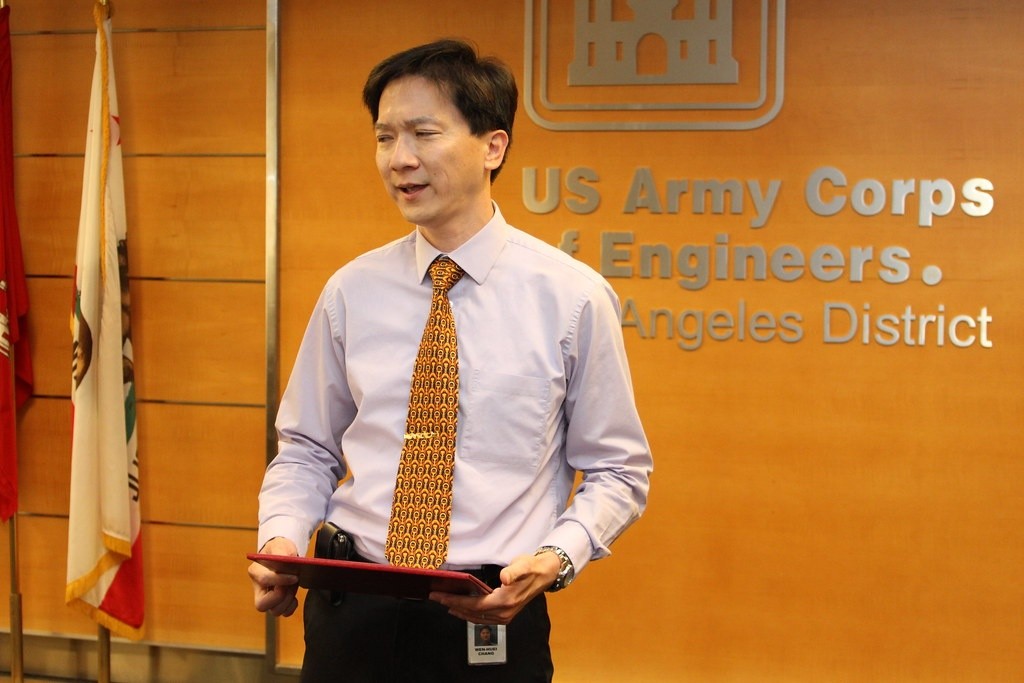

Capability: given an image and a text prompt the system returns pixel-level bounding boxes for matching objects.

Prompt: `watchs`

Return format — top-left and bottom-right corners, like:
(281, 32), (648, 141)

(535, 546), (575, 593)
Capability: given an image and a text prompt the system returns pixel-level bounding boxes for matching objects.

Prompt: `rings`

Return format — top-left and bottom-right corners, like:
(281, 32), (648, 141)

(481, 614), (485, 619)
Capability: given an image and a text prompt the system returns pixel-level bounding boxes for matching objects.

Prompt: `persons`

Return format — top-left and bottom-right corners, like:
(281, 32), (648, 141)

(248, 36), (654, 683)
(475, 627), (497, 646)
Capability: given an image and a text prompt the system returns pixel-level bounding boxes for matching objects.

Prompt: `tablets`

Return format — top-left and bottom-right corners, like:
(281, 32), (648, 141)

(247, 552), (493, 596)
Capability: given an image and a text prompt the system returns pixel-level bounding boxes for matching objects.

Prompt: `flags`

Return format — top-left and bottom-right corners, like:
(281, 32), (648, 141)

(0, 0), (34, 522)
(65, 0), (147, 642)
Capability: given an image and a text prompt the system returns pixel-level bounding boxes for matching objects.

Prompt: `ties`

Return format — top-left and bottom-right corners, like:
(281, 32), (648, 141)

(383, 256), (465, 569)
